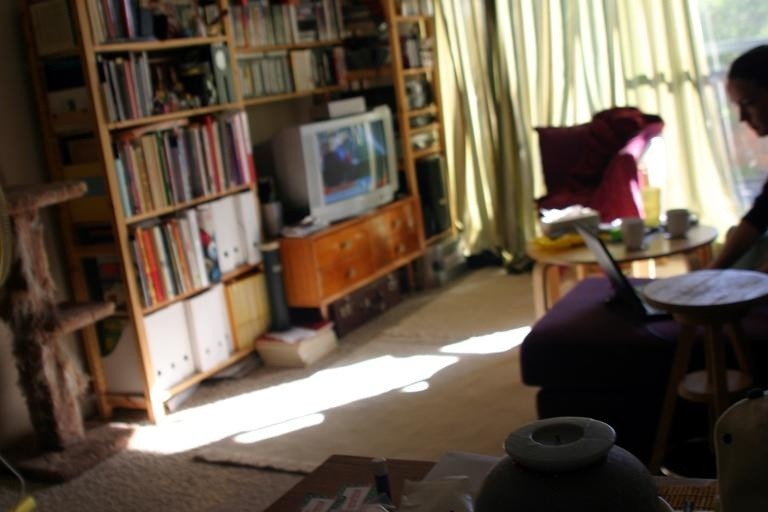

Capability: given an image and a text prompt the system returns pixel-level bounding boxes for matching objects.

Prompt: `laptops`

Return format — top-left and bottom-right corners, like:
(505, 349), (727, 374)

(574, 220), (671, 321)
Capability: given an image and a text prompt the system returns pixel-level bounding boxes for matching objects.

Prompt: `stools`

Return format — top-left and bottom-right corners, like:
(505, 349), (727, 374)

(520, 277), (768, 468)
(644, 269), (768, 456)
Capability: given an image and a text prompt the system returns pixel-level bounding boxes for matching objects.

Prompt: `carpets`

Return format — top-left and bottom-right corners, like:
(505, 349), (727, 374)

(192, 267), (574, 476)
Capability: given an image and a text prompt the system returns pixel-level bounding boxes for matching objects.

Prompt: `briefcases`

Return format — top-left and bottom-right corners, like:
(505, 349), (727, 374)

(330, 269), (402, 337)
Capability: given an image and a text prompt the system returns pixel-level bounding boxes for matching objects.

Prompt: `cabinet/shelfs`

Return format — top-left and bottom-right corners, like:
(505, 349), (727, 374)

(25, 2), (272, 419)
(276, 192), (426, 333)
(228, 0), (350, 109)
(333, 0), (459, 287)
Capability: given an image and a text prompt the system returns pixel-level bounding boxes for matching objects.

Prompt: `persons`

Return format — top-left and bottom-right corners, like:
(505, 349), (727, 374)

(666, 42), (768, 378)
(323, 128), (369, 187)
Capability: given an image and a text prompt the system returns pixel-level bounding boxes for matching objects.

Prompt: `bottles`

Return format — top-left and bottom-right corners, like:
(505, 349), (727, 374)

(372, 456), (391, 505)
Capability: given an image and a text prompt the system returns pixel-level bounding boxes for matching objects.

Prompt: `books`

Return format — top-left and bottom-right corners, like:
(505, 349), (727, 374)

(233, 1), (340, 100)
(31, 2), (262, 350)
(215, 321), (339, 380)
(338, 1), (440, 156)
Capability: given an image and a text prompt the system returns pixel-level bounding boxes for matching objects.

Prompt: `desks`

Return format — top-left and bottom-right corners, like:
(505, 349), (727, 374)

(263, 455), (723, 512)
(528, 225), (717, 319)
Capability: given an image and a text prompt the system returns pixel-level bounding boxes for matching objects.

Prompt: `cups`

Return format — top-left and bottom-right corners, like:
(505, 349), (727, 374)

(619, 217), (643, 250)
(666, 208), (688, 239)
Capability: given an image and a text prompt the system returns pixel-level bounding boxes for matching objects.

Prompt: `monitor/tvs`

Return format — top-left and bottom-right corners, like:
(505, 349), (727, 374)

(270, 104), (401, 225)
